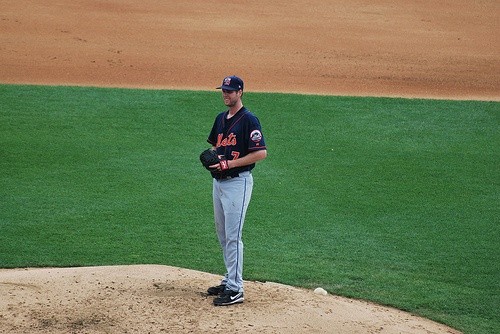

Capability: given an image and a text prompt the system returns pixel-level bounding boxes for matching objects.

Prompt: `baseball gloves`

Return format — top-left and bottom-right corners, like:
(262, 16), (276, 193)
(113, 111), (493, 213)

(197, 149), (226, 178)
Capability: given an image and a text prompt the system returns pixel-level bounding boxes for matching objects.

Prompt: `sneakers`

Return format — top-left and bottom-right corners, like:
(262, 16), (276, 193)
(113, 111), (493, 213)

(213, 289), (244, 306)
(207, 285), (226, 295)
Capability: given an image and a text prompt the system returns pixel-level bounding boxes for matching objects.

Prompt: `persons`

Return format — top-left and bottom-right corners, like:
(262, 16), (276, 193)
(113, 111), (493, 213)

(200, 77), (267, 306)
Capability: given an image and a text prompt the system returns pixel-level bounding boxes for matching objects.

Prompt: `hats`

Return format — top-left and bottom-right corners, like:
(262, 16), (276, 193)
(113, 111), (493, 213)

(216, 75), (243, 91)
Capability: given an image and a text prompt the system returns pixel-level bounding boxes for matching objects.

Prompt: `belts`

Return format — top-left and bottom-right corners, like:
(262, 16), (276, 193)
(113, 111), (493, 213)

(223, 174), (239, 178)
